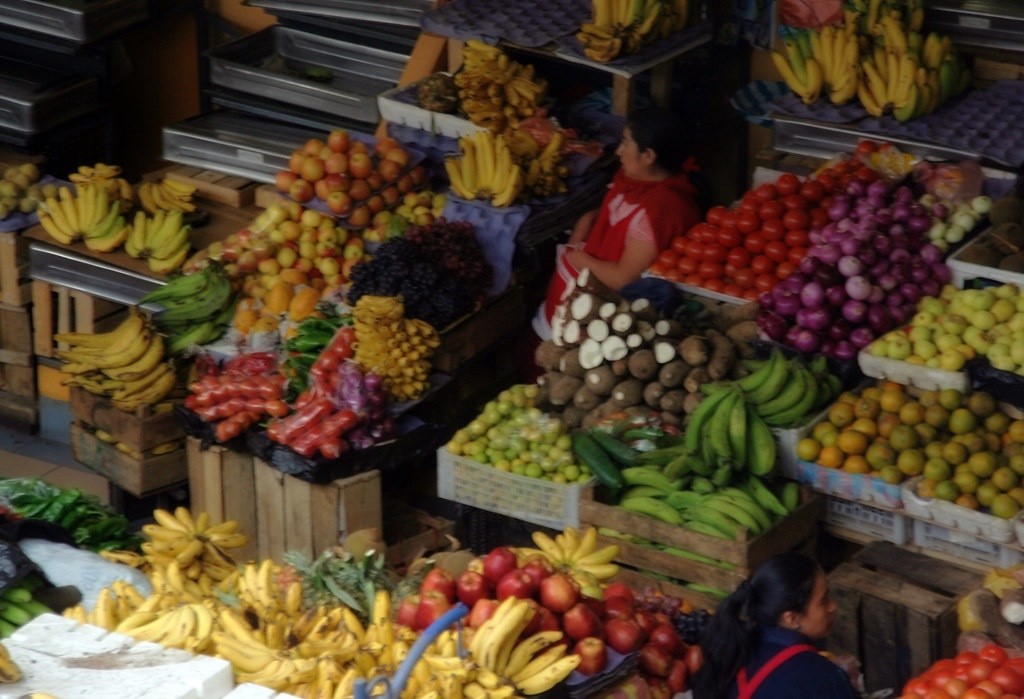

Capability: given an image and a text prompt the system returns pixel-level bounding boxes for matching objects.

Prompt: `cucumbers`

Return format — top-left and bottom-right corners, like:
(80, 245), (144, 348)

(570, 425), (644, 487)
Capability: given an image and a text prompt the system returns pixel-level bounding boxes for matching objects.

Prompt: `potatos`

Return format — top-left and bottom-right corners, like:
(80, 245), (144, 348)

(535, 267), (758, 431)
(954, 568), (1024, 663)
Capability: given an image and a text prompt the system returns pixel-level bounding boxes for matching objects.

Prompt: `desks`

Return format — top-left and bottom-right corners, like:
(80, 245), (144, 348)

(445, 34), (712, 118)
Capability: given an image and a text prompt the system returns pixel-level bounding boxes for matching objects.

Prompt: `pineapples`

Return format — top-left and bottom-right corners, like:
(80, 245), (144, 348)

(300, 550), (337, 610)
(342, 550), (388, 629)
(390, 556), (437, 619)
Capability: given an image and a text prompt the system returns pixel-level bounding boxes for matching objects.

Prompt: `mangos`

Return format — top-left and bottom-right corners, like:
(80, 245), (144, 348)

(232, 278), (338, 347)
(0, 164), (60, 221)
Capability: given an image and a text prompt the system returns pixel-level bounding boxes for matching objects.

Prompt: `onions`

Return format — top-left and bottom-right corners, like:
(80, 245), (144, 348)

(758, 179), (991, 358)
(337, 364), (394, 448)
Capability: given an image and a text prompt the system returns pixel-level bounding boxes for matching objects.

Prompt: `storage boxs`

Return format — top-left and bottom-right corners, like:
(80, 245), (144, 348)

(185, 437), (258, 562)
(381, 500), (456, 576)
(0, 0), (429, 186)
(376, 72), (486, 138)
(253, 458), (382, 567)
(912, 518), (1024, 570)
(823, 539), (987, 699)
(575, 474), (823, 614)
(826, 495), (913, 546)
(31, 280), (128, 364)
(791, 458), (918, 509)
(0, 613), (235, 699)
(944, 235), (1024, 291)
(857, 332), (970, 395)
(67, 387), (188, 497)
(900, 475), (1024, 545)
(428, 291), (528, 373)
(436, 444), (598, 531)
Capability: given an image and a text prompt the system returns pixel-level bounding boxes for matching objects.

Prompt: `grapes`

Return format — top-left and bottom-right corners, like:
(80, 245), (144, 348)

(632, 587), (685, 611)
(347, 218), (494, 330)
(675, 609), (710, 638)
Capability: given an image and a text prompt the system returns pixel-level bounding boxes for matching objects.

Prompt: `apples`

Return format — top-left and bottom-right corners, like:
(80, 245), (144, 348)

(870, 284), (1024, 375)
(184, 130), (445, 298)
(397, 545), (704, 698)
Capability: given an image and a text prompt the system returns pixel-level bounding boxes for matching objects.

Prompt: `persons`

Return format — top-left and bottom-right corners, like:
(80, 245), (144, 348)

(693, 552), (861, 699)
(487, 107), (698, 386)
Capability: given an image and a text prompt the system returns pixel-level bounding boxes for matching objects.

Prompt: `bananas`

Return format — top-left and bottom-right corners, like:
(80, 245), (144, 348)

(773, 0), (969, 120)
(355, 296), (441, 397)
(36, 163), (238, 461)
(445, 41), (572, 205)
(619, 345), (844, 570)
(0, 507), (619, 698)
(576, 0), (693, 64)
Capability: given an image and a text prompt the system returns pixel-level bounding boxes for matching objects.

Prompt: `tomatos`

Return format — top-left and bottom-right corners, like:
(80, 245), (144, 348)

(187, 353), (291, 441)
(268, 329), (357, 457)
(900, 643), (1024, 699)
(443, 381), (592, 487)
(653, 141), (991, 301)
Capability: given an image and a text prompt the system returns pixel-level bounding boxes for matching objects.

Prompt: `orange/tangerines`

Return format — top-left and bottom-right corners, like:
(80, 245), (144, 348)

(797, 380), (1024, 523)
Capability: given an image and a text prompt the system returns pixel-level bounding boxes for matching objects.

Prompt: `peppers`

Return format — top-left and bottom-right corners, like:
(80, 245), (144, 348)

(285, 316), (353, 392)
(1, 477), (145, 554)
